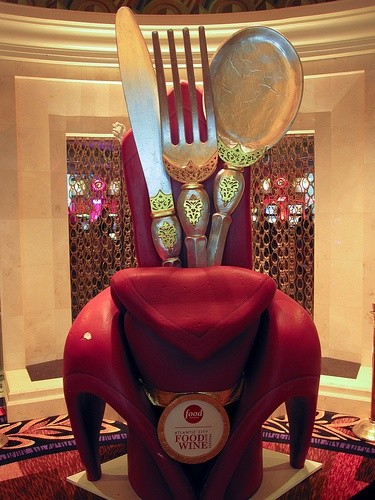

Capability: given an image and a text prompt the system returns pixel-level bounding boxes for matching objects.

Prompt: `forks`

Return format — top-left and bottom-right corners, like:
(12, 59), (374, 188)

(151, 25), (219, 268)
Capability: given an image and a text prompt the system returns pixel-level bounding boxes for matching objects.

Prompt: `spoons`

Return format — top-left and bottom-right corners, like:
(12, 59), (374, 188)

(202, 26), (304, 266)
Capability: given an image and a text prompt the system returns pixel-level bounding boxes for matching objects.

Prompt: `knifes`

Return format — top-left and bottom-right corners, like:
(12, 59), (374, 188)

(115, 6), (182, 268)
